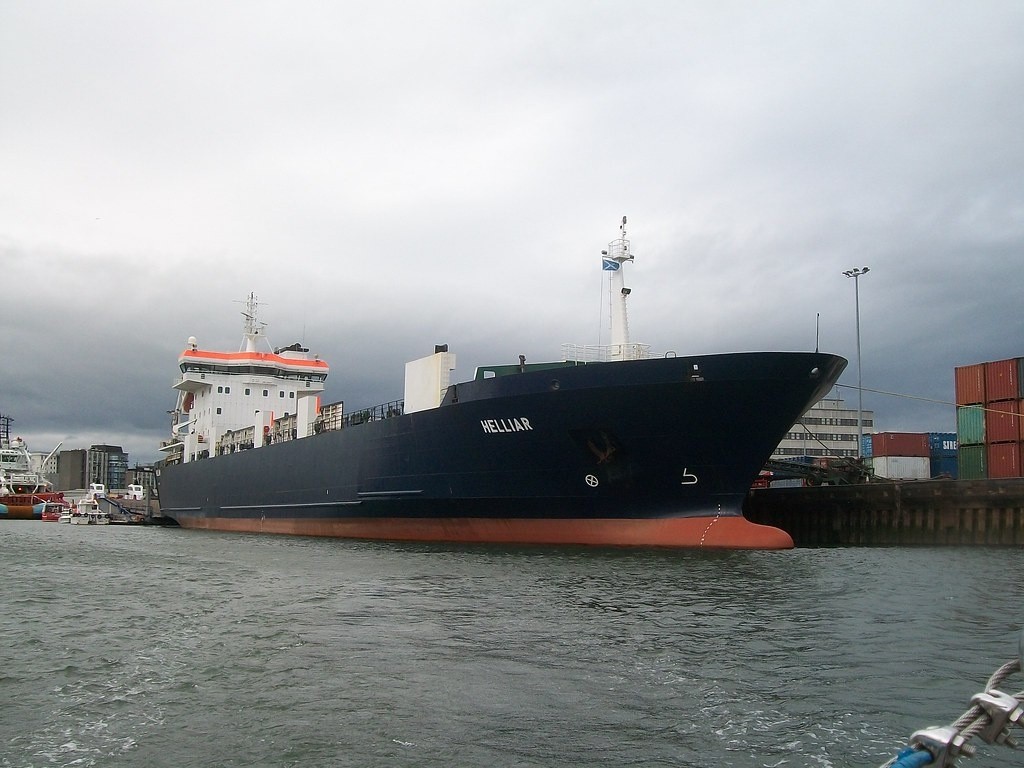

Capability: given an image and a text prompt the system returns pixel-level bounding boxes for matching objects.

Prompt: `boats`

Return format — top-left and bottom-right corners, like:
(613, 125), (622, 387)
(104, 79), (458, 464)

(0, 414), (70, 519)
(153, 214), (849, 550)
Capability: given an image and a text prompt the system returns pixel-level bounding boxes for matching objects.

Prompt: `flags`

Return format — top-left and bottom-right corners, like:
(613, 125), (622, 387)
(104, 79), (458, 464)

(603, 260), (619, 271)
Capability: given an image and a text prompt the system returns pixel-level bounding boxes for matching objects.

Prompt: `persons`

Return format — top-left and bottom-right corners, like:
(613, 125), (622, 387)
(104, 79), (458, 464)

(47, 508), (52, 512)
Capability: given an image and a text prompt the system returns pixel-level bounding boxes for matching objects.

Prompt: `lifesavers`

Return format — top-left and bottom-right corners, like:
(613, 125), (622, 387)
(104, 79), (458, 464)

(74, 513), (77, 517)
(105, 514), (109, 519)
(98, 515), (101, 519)
(140, 518), (145, 522)
(77, 513), (81, 517)
(84, 513), (88, 517)
(91, 515), (95, 518)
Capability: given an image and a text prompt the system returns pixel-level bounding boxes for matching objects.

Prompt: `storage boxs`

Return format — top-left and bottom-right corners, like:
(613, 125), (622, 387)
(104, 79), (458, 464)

(751, 357), (1024, 488)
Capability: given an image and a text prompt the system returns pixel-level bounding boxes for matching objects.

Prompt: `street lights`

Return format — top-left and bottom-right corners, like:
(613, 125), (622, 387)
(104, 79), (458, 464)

(841, 267), (871, 461)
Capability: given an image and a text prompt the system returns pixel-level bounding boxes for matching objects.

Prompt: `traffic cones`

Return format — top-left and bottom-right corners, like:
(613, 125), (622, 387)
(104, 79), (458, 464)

(864, 474), (870, 485)
